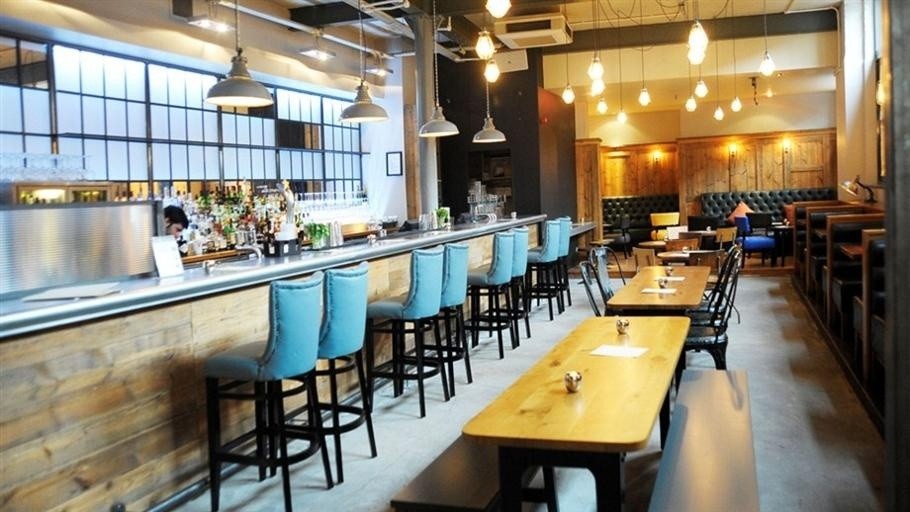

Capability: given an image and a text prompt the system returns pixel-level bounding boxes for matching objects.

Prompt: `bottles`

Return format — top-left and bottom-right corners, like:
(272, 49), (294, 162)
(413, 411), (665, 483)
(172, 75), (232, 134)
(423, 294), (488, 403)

(335, 220), (345, 245)
(116, 183), (281, 259)
(367, 234), (376, 246)
(204, 260), (216, 275)
(327, 222), (337, 248)
(617, 319), (628, 332)
(565, 372), (582, 391)
(666, 267), (674, 276)
(19, 195), (60, 206)
(429, 209), (439, 230)
(659, 279), (668, 289)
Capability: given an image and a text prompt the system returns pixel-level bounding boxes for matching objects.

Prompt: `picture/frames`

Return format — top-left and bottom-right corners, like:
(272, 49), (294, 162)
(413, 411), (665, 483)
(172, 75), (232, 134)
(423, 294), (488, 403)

(386, 151), (402, 176)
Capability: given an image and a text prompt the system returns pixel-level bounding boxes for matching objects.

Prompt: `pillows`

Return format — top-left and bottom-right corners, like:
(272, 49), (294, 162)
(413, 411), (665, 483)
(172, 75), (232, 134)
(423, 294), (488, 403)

(727, 200), (754, 225)
(783, 204), (796, 225)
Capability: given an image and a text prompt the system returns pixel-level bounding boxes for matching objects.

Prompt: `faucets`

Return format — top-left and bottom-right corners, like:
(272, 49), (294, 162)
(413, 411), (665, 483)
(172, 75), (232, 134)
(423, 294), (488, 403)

(234, 245), (262, 260)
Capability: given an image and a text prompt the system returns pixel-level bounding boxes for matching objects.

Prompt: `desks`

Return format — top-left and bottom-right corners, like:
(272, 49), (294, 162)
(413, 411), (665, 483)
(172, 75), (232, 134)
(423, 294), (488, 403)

(462, 316), (691, 512)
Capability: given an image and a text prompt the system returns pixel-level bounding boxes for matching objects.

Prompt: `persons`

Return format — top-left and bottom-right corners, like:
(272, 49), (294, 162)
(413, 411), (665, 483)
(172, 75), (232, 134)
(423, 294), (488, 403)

(161, 206), (191, 242)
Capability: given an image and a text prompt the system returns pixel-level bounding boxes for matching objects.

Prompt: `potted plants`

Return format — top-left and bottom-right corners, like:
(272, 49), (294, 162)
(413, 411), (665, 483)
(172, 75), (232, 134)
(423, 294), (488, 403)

(436, 209), (448, 230)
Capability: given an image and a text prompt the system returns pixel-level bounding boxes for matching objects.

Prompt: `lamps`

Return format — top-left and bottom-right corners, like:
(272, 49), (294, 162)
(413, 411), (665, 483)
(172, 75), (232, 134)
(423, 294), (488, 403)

(419, 2), (460, 139)
(333, 2), (394, 125)
(839, 175), (878, 202)
(473, 1), (776, 123)
(471, 84), (507, 144)
(203, 2), (277, 109)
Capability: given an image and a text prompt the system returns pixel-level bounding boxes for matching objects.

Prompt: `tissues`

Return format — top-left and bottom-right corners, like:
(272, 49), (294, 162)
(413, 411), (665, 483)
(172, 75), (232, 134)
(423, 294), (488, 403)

(264, 223), (300, 256)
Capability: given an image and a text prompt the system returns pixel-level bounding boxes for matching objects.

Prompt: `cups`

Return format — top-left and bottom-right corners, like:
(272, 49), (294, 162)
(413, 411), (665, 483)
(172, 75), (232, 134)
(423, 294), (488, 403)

(511, 211), (517, 218)
(467, 182), (498, 214)
(441, 206), (451, 225)
(418, 213), (428, 232)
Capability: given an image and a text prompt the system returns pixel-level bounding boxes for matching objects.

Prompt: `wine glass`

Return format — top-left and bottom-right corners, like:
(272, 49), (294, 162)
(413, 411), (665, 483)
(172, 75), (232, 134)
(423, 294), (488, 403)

(292, 190), (370, 225)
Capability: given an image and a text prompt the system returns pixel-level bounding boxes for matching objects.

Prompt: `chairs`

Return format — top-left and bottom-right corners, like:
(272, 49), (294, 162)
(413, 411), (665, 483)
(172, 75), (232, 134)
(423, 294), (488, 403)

(466, 230), (518, 359)
(366, 244), (450, 419)
(529, 214), (571, 313)
(205, 271), (337, 512)
(279, 259), (378, 486)
(527, 218), (563, 321)
(397, 244), (473, 399)
(488, 225), (533, 348)
(579, 212), (775, 369)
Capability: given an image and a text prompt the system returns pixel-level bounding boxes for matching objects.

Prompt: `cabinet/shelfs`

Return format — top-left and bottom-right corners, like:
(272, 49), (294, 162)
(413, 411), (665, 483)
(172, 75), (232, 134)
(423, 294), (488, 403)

(482, 149), (513, 215)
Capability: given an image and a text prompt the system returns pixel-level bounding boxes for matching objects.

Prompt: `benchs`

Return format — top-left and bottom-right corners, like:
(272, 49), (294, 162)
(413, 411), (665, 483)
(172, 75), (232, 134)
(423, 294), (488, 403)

(602, 194), (679, 241)
(792, 200), (887, 380)
(700, 187), (837, 249)
(648, 370), (759, 512)
